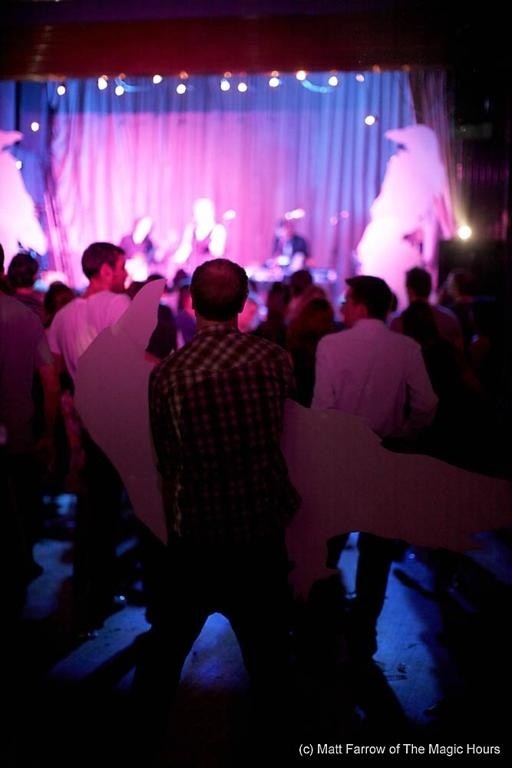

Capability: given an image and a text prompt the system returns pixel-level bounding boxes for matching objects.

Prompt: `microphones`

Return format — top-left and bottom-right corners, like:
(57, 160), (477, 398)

(285, 208), (304, 220)
